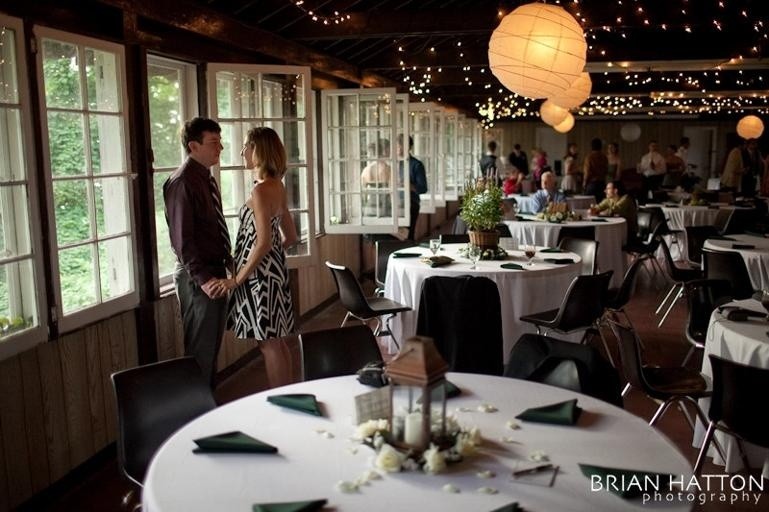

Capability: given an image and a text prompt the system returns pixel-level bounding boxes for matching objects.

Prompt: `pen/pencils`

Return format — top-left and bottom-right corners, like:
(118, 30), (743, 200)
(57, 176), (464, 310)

(513, 464), (552, 476)
(549, 466), (559, 487)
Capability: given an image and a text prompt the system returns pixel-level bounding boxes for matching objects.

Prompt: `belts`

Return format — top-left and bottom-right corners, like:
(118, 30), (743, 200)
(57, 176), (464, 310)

(211, 258), (230, 264)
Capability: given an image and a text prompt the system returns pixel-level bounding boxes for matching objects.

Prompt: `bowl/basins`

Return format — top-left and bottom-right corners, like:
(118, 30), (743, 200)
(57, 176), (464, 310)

(667, 192), (690, 202)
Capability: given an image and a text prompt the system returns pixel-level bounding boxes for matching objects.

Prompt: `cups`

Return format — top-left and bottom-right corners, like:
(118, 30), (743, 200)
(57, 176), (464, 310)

(760, 287), (769, 314)
(590, 203), (600, 216)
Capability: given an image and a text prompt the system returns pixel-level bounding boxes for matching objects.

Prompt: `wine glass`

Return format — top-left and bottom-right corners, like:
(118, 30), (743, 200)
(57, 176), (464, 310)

(525, 242), (539, 267)
(468, 244), (481, 271)
(429, 238), (440, 258)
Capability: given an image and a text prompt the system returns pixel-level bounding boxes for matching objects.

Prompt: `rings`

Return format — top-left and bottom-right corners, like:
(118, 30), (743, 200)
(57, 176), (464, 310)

(217, 281), (221, 286)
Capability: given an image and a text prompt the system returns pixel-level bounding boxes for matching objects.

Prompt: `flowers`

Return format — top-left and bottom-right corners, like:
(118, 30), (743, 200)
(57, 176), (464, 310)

(357, 406), (486, 476)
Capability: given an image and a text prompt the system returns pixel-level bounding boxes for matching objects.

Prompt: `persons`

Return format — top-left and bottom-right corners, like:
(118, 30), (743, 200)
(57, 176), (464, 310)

(163, 117), (233, 394)
(479, 136), (769, 251)
(396, 133), (427, 240)
(361, 139), (391, 277)
(207, 126), (301, 388)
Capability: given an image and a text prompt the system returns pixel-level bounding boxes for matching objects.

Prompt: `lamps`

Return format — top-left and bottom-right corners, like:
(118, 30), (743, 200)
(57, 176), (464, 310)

(488, 0), (591, 133)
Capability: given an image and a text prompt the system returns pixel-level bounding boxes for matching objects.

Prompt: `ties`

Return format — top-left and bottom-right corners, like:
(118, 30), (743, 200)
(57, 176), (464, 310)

(207, 178), (232, 255)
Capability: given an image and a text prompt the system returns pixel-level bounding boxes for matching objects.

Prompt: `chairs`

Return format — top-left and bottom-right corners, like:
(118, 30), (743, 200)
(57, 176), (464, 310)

(691, 353), (769, 483)
(108, 353), (217, 512)
(299, 324), (385, 381)
(610, 319), (727, 464)
(503, 333), (625, 412)
(325, 167), (769, 372)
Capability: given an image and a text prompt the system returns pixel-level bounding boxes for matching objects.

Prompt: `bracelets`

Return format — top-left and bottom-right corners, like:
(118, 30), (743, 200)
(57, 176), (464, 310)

(233, 277), (239, 288)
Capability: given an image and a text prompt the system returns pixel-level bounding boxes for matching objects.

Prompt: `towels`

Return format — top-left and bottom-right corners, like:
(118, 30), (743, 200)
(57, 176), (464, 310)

(267, 393), (322, 414)
(415, 380), (461, 403)
(514, 397), (583, 426)
(577, 462), (676, 500)
(357, 369), (387, 388)
(252, 497), (327, 511)
(191, 429), (278, 454)
